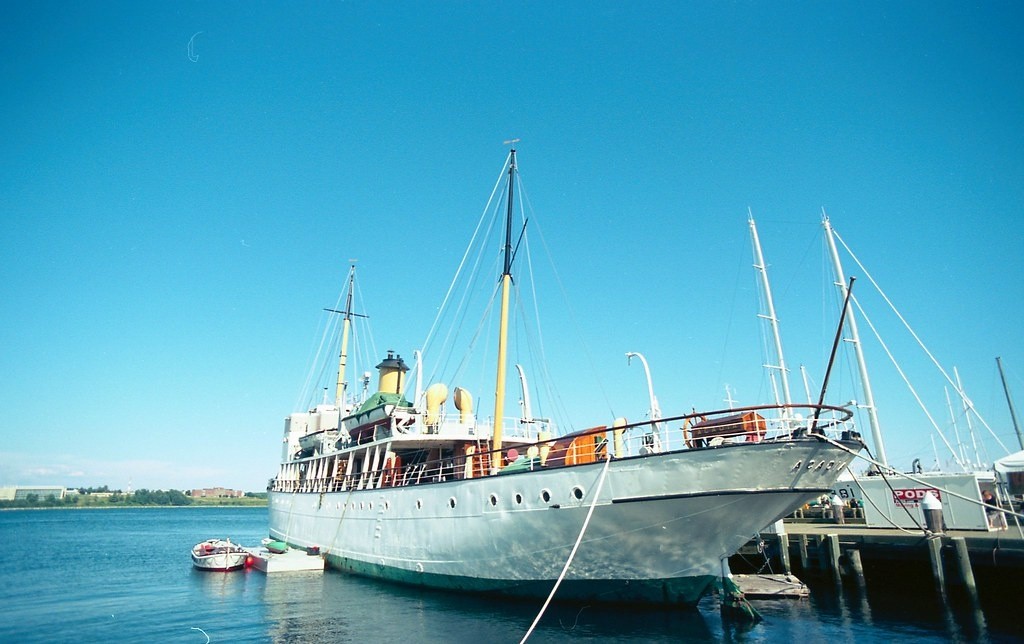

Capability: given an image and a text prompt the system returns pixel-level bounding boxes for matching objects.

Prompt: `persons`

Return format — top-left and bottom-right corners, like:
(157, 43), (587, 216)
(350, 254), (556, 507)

(850, 498), (863, 508)
(982, 490), (996, 527)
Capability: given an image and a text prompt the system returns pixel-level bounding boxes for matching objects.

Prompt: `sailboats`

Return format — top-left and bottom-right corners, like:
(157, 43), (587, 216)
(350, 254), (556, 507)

(268, 134), (1023, 614)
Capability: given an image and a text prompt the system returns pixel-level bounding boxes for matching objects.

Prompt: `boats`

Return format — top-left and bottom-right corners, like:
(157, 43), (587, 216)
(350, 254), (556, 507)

(297, 428), (339, 451)
(190, 537), (248, 572)
(262, 539), (286, 553)
(341, 391), (421, 445)
